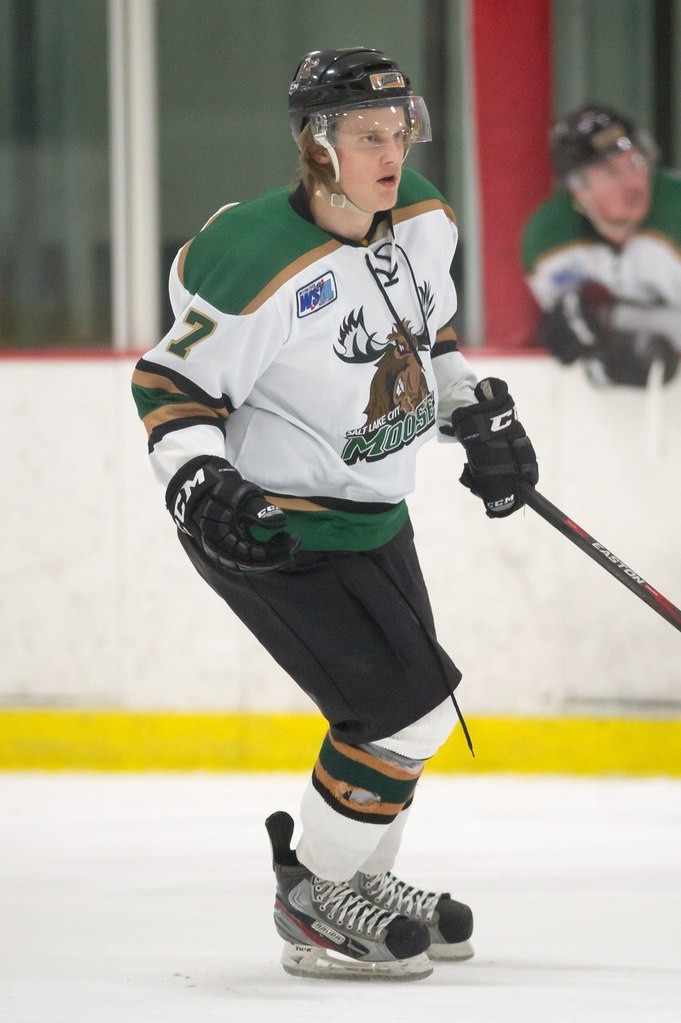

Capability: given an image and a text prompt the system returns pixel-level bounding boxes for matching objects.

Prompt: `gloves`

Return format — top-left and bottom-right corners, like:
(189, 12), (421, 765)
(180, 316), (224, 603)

(596, 330), (679, 388)
(165, 455), (302, 578)
(534, 279), (598, 364)
(438, 378), (539, 518)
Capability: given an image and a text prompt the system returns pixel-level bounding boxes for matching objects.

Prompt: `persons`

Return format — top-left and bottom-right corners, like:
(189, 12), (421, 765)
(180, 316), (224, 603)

(518, 102), (681, 388)
(131, 46), (538, 962)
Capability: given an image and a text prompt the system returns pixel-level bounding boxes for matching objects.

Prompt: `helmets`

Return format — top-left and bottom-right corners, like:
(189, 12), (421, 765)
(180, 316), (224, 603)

(548, 102), (658, 191)
(287, 46), (433, 149)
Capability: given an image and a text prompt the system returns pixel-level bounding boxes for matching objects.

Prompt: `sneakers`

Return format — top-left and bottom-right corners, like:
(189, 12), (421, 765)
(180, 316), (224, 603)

(264, 811), (434, 983)
(349, 871), (475, 962)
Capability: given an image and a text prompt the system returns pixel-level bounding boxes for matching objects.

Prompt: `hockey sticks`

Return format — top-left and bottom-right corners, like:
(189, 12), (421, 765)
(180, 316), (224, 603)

(517, 475), (681, 633)
